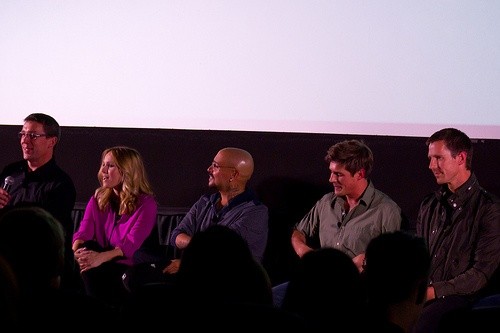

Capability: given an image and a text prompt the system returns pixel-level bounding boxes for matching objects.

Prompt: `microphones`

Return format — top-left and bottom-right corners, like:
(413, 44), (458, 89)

(3, 176), (15, 193)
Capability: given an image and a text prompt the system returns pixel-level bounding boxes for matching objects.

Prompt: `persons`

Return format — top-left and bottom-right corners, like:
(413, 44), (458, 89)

(271, 139), (401, 308)
(415, 127), (500, 304)
(162, 147), (268, 274)
(72, 146), (158, 273)
(0, 200), (500, 333)
(0, 113), (79, 261)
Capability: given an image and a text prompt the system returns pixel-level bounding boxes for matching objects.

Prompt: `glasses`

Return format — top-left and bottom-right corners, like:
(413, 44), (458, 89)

(17, 132), (55, 142)
(211, 161), (239, 175)
